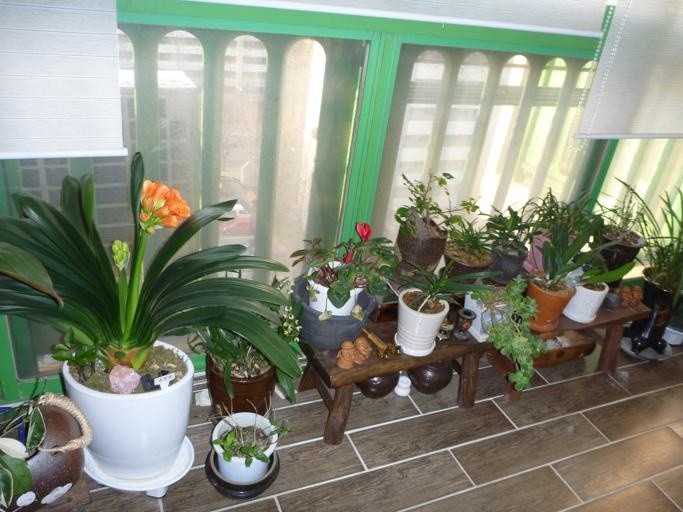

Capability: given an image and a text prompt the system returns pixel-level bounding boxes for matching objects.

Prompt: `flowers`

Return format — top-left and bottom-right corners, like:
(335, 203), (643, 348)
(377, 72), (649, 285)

(0, 149), (302, 407)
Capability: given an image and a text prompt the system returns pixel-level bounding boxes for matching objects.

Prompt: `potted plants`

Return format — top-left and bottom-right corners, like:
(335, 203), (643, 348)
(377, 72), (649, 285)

(185, 266), (303, 418)
(0, 392), (86, 512)
(201, 390), (294, 503)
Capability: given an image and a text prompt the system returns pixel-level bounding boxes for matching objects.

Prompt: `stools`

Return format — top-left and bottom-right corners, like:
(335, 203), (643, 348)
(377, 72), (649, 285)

(293, 325), (488, 447)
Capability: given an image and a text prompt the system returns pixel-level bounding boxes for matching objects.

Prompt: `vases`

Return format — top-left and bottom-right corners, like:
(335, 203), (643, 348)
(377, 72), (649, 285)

(59, 340), (197, 501)
